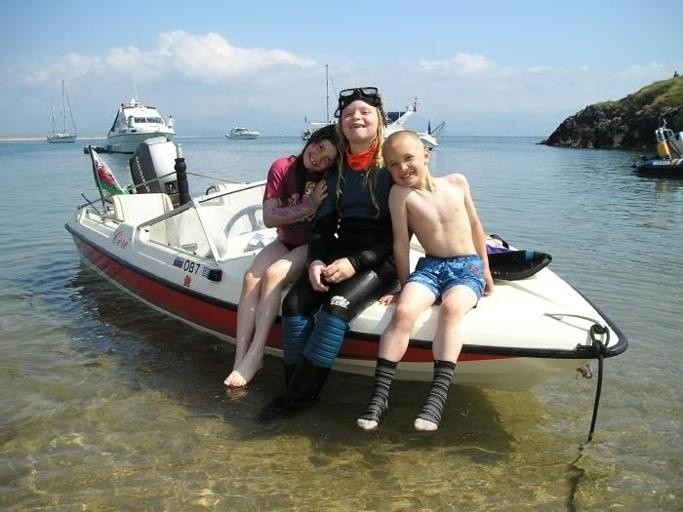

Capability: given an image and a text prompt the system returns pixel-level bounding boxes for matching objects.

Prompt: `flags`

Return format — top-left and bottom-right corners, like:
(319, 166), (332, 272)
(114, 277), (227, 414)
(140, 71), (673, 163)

(91, 149), (130, 195)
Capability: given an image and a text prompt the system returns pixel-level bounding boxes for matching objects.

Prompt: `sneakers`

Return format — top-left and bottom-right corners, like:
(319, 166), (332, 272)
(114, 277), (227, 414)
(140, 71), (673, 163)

(260, 388), (318, 422)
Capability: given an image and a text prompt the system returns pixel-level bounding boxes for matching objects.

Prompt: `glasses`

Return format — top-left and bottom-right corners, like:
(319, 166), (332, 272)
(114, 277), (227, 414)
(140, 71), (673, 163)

(339, 87), (378, 112)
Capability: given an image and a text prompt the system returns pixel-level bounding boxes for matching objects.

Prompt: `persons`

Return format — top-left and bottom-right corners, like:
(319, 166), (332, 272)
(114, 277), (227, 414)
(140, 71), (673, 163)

(224, 124), (340, 399)
(356, 130), (493, 431)
(255, 87), (414, 423)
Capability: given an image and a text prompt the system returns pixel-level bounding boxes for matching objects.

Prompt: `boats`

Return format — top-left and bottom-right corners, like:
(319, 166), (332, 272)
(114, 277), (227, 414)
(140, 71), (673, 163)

(631, 120), (683, 174)
(46, 78), (260, 153)
(304, 63), (417, 137)
(64, 144), (629, 366)
